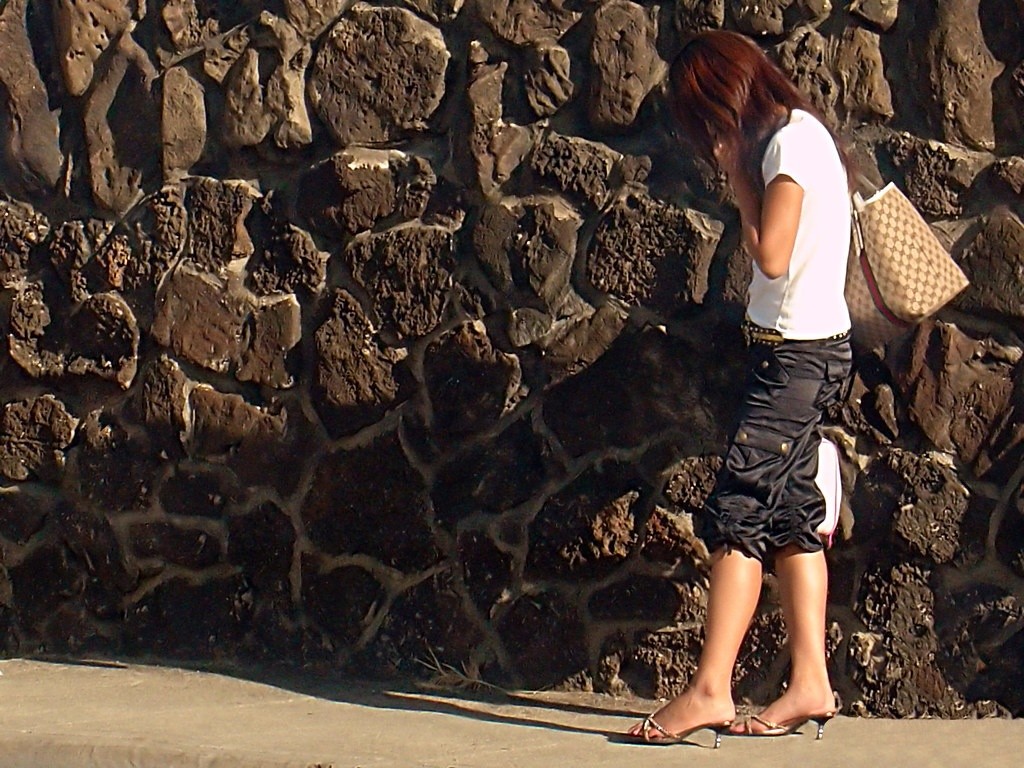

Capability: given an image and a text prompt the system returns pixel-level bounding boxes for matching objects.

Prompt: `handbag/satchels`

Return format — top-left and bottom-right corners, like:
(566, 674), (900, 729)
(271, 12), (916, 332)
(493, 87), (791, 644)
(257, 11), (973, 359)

(843, 180), (970, 349)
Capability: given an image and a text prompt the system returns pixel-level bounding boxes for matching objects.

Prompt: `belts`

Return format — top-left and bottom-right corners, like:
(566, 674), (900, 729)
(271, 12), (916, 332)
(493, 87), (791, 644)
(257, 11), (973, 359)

(742, 321), (849, 348)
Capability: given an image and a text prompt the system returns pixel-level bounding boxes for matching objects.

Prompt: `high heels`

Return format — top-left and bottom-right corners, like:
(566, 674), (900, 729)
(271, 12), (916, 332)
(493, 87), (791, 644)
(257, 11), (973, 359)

(608, 701), (733, 749)
(724, 710), (835, 740)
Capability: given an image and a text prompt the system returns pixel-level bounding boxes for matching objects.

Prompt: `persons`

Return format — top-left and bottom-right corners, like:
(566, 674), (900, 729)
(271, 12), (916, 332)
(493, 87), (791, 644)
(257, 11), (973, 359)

(628, 29), (856, 749)
